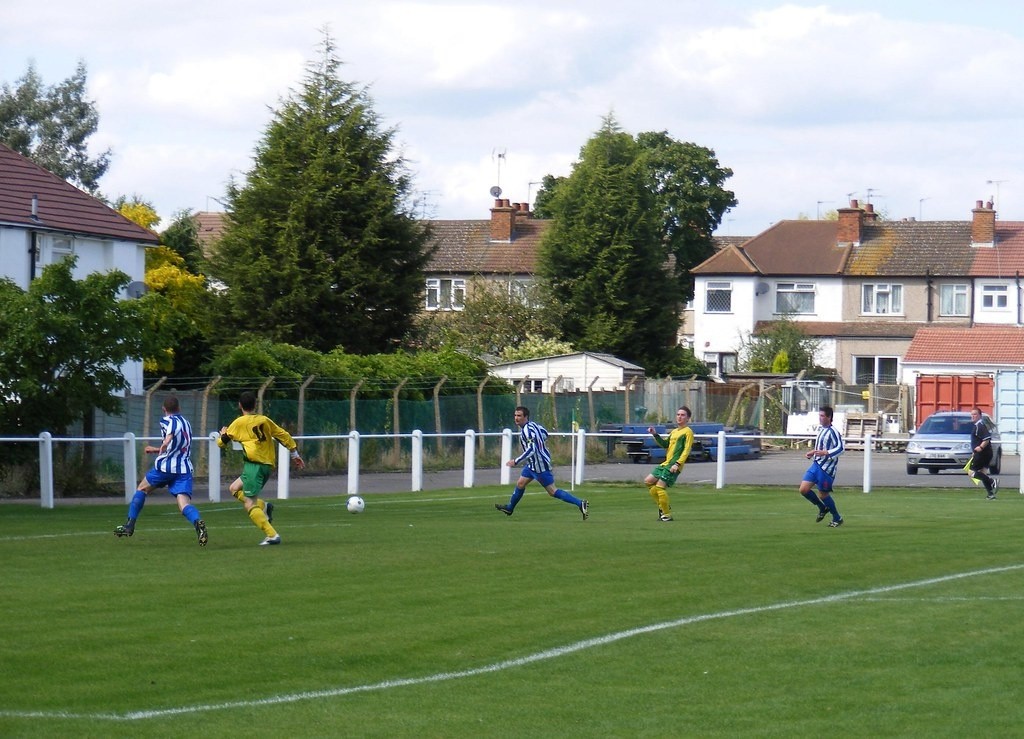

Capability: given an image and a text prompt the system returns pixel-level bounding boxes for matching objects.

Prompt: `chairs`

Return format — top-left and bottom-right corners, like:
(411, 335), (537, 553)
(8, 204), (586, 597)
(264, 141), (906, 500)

(959, 424), (973, 432)
(934, 422), (953, 430)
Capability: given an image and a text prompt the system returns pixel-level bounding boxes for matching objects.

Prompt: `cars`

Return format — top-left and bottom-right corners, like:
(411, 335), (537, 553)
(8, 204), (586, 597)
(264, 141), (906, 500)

(905, 411), (1002, 475)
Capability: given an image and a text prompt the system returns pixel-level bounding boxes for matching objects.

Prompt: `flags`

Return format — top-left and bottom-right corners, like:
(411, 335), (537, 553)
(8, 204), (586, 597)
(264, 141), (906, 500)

(965, 457), (980, 484)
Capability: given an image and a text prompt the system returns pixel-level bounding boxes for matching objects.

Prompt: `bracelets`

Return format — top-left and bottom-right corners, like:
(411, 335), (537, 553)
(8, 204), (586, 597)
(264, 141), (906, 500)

(291, 451), (299, 458)
(979, 446), (983, 450)
(674, 463), (679, 467)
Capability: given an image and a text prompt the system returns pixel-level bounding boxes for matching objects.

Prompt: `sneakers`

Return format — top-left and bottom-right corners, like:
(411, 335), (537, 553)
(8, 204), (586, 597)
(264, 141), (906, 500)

(991, 477), (999, 494)
(659, 505), (671, 520)
(816, 506), (830, 522)
(579, 500), (589, 520)
(258, 533), (284, 546)
(494, 503), (513, 516)
(827, 516), (844, 528)
(660, 515), (673, 522)
(984, 495), (995, 501)
(262, 501), (272, 523)
(193, 519), (208, 547)
(113, 517), (135, 537)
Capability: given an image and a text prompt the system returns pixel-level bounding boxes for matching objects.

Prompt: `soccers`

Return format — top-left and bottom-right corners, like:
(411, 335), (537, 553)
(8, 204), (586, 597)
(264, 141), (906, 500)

(346, 495), (366, 514)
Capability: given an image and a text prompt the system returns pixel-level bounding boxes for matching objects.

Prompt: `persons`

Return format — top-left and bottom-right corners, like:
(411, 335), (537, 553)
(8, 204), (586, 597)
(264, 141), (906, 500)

(217, 392), (304, 545)
(495, 407), (589, 520)
(644, 406), (693, 521)
(800, 406), (843, 527)
(968, 407), (1000, 500)
(113, 397), (208, 546)
